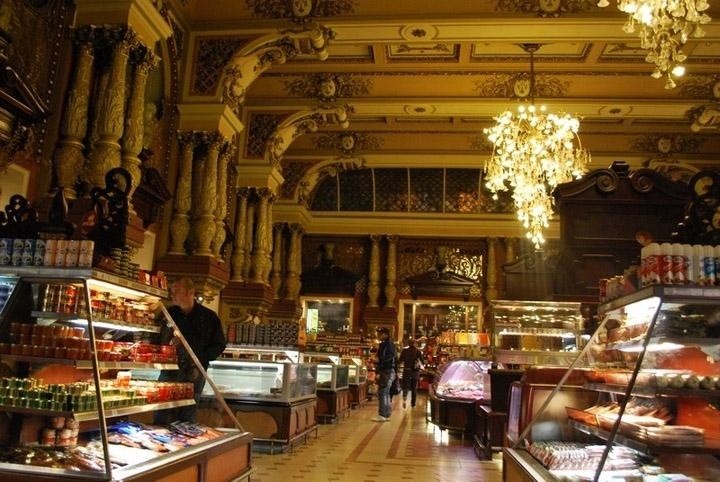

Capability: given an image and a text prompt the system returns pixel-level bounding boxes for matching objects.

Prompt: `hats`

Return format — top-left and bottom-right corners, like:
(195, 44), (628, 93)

(376, 326), (389, 333)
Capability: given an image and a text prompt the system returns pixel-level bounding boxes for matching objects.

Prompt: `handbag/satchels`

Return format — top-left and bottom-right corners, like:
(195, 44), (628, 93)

(390, 382), (401, 395)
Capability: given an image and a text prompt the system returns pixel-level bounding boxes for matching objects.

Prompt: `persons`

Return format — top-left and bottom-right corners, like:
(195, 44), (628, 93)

(367, 327), (397, 421)
(398, 339), (425, 407)
(149, 276), (226, 427)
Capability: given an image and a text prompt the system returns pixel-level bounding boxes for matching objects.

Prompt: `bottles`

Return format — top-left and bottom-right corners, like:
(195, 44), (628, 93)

(597, 242), (720, 306)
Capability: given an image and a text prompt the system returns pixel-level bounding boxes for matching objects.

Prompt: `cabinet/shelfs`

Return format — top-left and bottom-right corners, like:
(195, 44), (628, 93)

(502, 284), (720, 481)
(1, 267), (256, 482)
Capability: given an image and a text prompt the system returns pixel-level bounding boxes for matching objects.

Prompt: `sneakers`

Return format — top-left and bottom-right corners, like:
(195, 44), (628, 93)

(370, 415), (391, 422)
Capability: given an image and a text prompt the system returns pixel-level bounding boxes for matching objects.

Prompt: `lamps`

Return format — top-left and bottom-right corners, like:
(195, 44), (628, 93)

(597, 0), (712, 90)
(482, 43), (592, 252)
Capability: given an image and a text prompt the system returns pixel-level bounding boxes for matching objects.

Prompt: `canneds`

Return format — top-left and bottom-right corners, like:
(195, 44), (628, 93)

(112, 247), (141, 281)
(0, 322), (122, 364)
(0, 238), (96, 267)
(37, 281), (133, 327)
(0, 377), (196, 412)
